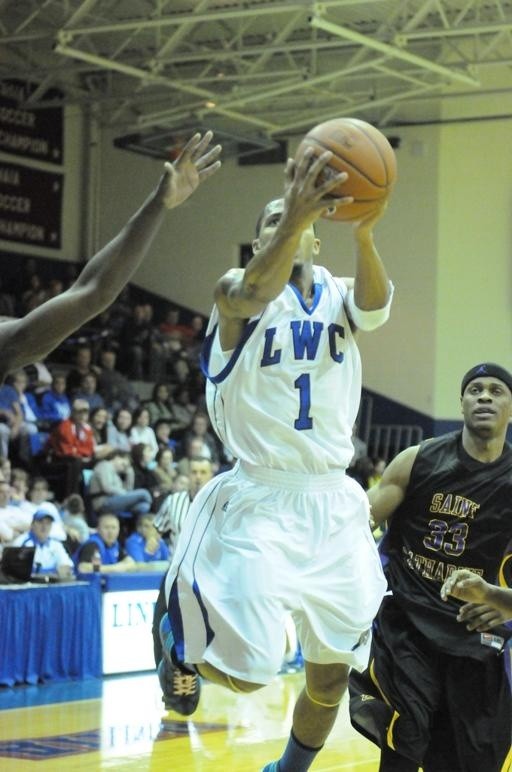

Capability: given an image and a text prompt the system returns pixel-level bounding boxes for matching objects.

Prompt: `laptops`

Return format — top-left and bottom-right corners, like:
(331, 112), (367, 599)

(1, 547), (35, 581)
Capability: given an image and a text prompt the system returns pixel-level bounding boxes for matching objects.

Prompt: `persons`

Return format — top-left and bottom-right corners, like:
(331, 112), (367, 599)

(151, 149), (395, 770)
(152, 457), (216, 671)
(440, 567), (512, 622)
(1, 128), (226, 382)
(1, 255), (391, 586)
(344, 360), (512, 771)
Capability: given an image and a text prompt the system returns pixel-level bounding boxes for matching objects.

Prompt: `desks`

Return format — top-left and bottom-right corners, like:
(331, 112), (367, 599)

(1, 570), (166, 688)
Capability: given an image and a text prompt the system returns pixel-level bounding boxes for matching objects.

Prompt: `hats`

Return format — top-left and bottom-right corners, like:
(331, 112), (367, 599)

(34, 510), (54, 522)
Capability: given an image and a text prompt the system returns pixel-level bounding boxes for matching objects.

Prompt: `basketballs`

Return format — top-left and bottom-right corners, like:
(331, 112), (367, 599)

(296, 118), (397, 222)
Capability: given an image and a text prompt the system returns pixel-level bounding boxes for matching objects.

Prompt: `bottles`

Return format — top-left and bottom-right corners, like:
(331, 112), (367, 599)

(91, 548), (102, 574)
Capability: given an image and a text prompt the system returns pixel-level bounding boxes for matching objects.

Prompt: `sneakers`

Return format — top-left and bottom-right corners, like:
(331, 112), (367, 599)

(157, 614), (200, 715)
